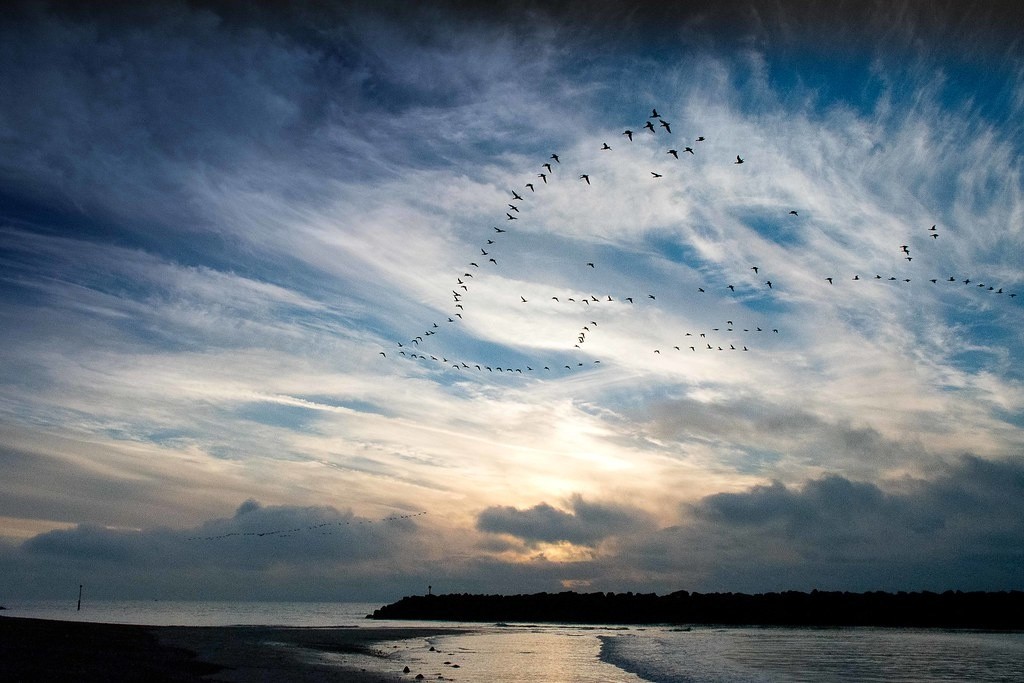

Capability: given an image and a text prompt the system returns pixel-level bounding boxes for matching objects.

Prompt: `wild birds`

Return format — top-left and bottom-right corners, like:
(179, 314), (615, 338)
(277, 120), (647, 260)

(649, 109), (661, 118)
(696, 137), (705, 141)
(183, 511), (427, 540)
(623, 130), (633, 141)
(379, 142), (1017, 373)
(659, 119), (671, 133)
(643, 121), (655, 133)
